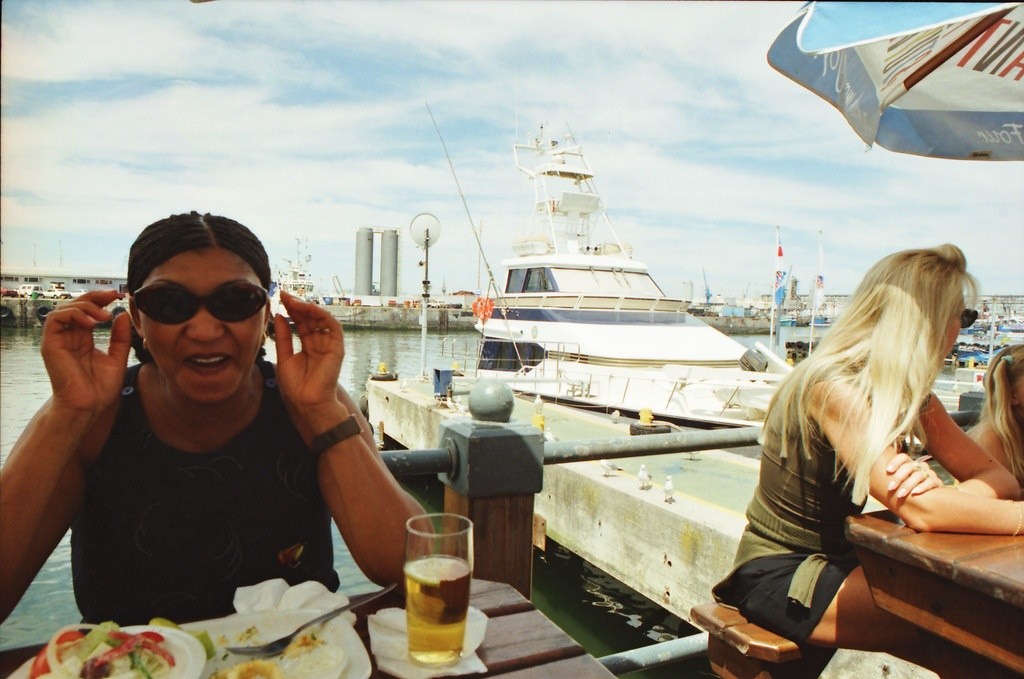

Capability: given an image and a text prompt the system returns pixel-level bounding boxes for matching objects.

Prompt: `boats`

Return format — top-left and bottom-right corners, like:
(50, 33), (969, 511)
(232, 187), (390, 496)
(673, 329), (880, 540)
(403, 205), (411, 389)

(270, 226), (326, 326)
(946, 297), (1023, 366)
(422, 110), (832, 432)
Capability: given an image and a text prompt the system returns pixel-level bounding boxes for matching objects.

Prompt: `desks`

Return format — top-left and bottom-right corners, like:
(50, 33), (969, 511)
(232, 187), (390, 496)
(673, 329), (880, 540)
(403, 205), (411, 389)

(844, 508), (1024, 679)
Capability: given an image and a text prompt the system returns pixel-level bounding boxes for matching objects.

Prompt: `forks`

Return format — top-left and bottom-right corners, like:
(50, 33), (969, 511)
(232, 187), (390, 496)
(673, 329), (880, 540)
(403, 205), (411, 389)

(225, 582), (398, 656)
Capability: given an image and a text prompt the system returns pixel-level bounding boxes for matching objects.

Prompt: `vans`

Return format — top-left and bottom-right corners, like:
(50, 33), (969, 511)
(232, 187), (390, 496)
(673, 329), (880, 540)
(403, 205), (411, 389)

(17, 285), (43, 298)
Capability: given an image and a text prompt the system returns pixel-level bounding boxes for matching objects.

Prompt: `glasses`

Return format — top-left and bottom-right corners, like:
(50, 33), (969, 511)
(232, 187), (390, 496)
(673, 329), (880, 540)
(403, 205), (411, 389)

(960, 309), (978, 328)
(132, 283), (269, 324)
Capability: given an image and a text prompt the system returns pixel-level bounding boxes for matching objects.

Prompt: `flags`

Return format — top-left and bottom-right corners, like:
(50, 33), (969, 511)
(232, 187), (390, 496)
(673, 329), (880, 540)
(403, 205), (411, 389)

(813, 241), (823, 307)
(773, 237), (785, 306)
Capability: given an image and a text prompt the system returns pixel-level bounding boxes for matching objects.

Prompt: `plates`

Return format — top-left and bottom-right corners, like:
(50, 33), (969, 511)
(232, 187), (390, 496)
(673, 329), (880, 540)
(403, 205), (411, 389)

(178, 610), (372, 679)
(4, 625), (207, 679)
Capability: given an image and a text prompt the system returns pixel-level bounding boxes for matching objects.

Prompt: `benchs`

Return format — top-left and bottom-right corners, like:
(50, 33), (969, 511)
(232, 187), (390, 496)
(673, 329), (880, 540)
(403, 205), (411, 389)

(689, 602), (837, 679)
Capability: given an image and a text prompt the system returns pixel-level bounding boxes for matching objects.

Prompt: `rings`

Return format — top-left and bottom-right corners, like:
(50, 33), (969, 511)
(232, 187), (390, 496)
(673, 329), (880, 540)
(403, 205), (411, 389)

(323, 329), (329, 333)
(312, 327), (319, 333)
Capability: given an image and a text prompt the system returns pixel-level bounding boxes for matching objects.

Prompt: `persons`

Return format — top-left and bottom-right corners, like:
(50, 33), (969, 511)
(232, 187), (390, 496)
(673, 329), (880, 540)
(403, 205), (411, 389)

(714, 244), (1024, 679)
(976, 344), (1023, 479)
(0, 213), (432, 639)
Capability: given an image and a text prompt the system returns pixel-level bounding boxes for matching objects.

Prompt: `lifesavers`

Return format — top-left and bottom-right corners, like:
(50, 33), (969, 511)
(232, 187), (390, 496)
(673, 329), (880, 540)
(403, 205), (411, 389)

(0, 305), (11, 320)
(785, 341), (1009, 353)
(110, 306), (126, 320)
(479, 298), (494, 320)
(36, 305), (52, 320)
(473, 298), (483, 316)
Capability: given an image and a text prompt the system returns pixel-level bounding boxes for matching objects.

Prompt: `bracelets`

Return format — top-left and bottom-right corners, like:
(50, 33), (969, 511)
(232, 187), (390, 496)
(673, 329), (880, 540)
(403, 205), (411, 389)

(314, 414), (362, 450)
(1013, 501), (1023, 536)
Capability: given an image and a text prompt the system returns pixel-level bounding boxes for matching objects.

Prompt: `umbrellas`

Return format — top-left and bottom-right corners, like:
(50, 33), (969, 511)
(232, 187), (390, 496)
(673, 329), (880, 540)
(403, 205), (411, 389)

(765, 1), (1024, 161)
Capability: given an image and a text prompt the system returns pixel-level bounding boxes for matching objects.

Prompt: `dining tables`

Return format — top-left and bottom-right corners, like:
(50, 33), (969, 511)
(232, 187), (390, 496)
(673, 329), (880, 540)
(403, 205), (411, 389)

(0, 572), (620, 679)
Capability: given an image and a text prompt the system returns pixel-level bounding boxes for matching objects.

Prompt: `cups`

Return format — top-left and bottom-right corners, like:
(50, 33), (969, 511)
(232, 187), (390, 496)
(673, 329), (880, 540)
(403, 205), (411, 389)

(403, 513), (474, 669)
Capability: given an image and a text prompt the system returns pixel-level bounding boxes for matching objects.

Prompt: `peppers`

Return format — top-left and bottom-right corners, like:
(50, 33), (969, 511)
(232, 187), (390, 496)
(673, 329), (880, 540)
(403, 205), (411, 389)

(28, 621), (175, 679)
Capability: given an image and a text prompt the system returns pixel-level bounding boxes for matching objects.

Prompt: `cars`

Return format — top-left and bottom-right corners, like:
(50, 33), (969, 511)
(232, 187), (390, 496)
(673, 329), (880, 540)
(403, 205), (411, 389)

(42, 288), (69, 299)
(1, 287), (17, 298)
